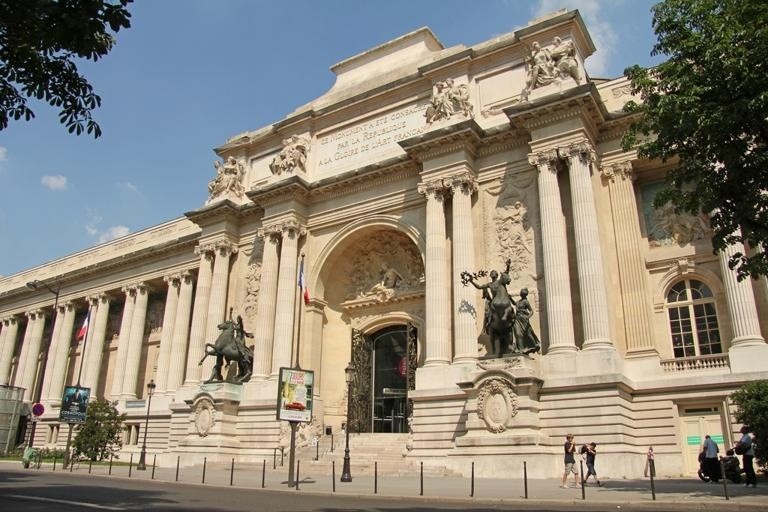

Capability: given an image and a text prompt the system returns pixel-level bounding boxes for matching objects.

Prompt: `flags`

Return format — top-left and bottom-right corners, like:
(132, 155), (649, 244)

(298, 261), (310, 304)
(78, 308), (92, 340)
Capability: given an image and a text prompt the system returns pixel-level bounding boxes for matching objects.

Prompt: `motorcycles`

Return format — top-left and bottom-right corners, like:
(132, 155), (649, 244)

(698, 450), (741, 484)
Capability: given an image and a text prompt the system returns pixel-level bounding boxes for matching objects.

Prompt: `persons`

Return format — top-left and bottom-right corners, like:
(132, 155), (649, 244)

(582, 441), (602, 487)
(733, 425), (758, 488)
(428, 81), (451, 123)
(560, 433), (582, 489)
(360, 262), (405, 298)
(225, 312), (251, 367)
(703, 434), (721, 484)
(205, 134), (306, 200)
(525, 41), (554, 88)
(446, 77), (475, 118)
(470, 258), (513, 327)
(549, 35), (580, 85)
(508, 287), (533, 351)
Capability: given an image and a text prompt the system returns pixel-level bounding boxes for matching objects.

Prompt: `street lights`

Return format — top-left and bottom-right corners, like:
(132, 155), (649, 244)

(136, 379), (156, 470)
(340, 361), (357, 482)
(23, 277), (63, 468)
(3, 387), (25, 456)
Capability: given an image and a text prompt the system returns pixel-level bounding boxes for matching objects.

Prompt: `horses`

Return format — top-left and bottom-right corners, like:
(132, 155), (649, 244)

(488, 271), (513, 358)
(198, 319), (253, 382)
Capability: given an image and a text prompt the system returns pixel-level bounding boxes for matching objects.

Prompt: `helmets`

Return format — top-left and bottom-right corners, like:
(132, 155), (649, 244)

(726, 449), (734, 456)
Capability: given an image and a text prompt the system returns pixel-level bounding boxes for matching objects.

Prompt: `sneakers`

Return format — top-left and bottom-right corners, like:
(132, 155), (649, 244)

(709, 479), (756, 488)
(562, 481), (607, 489)
